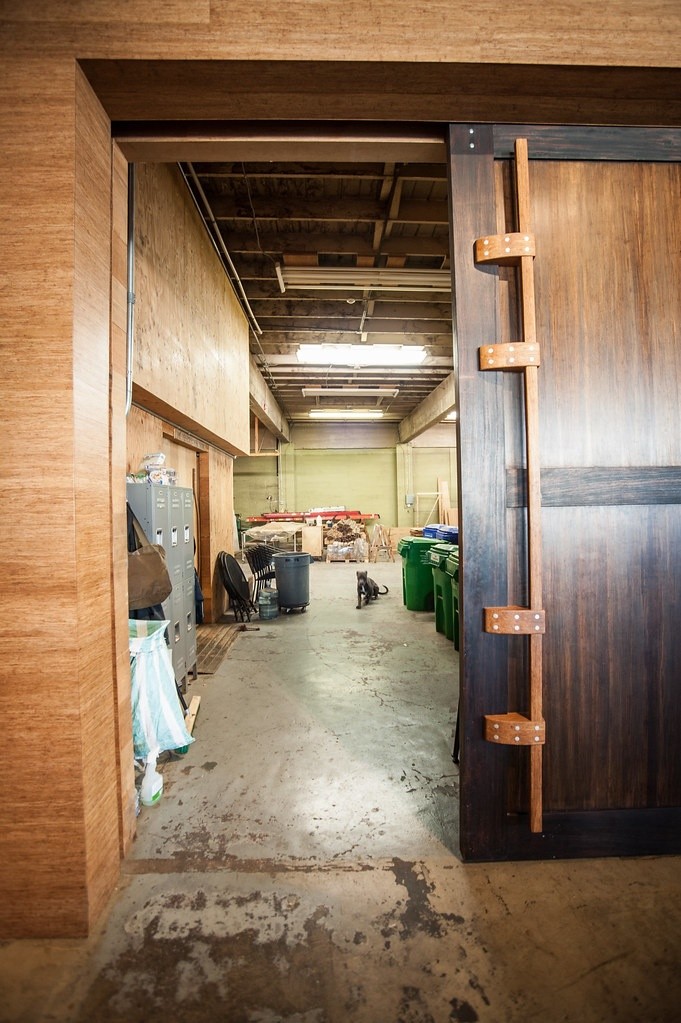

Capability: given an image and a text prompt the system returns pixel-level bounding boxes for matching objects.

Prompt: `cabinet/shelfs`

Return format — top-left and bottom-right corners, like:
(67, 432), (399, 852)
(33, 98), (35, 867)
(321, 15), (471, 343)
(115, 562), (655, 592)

(124, 483), (195, 684)
(302, 525), (323, 556)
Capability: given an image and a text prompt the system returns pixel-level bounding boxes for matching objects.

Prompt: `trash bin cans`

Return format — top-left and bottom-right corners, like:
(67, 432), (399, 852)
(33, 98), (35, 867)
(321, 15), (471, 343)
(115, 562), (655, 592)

(398, 536), (449, 613)
(422, 522), (459, 545)
(426, 543), (462, 652)
(272, 552), (311, 607)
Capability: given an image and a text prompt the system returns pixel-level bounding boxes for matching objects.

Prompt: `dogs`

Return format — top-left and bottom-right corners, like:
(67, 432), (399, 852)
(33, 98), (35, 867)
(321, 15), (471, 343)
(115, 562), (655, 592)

(356, 571), (389, 609)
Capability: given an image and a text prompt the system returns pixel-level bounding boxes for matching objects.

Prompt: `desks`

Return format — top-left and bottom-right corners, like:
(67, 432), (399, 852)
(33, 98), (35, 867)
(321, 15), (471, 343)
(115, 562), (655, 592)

(216, 551), (256, 622)
(240, 522), (307, 563)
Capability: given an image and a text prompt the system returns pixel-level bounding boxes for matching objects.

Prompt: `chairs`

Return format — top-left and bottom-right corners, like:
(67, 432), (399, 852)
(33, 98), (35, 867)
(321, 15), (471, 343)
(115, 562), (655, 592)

(243, 544), (285, 604)
